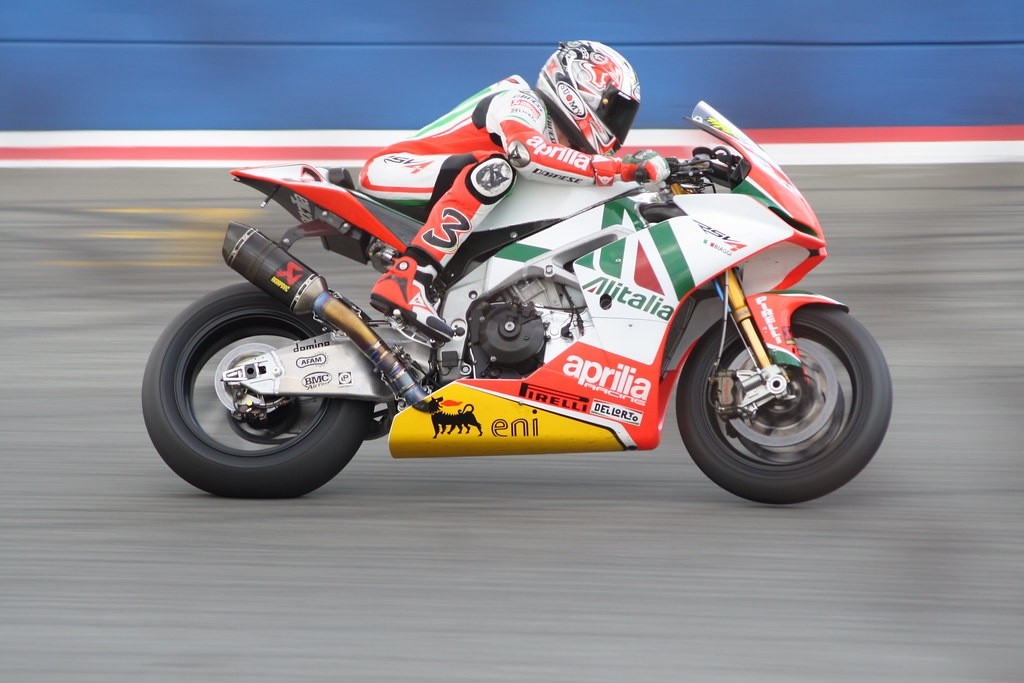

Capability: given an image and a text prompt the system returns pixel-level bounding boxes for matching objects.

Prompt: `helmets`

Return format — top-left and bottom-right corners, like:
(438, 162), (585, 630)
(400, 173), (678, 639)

(534, 40), (640, 156)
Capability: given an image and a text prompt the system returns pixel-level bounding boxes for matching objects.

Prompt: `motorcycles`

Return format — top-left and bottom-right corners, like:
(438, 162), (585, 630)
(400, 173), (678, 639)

(139, 95), (894, 506)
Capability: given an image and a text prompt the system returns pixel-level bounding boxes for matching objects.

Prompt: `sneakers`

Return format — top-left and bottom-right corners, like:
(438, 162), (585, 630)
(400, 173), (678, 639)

(369, 253), (453, 343)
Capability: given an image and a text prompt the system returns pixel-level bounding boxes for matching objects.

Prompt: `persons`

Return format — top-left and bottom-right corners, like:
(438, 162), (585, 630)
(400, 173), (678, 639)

(357, 40), (671, 347)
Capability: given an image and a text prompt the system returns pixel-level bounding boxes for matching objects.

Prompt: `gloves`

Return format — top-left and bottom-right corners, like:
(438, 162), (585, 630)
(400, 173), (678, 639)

(621, 148), (670, 182)
